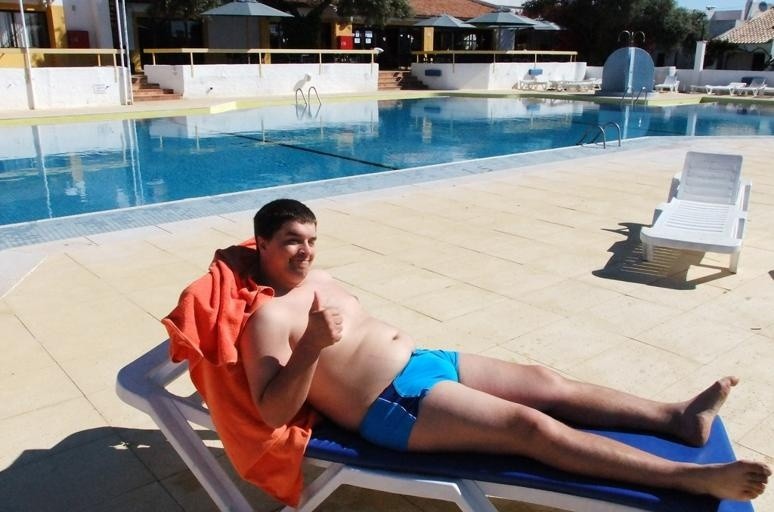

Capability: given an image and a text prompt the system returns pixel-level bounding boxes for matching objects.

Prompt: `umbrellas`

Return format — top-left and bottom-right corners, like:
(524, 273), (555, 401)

(413, 12), (566, 49)
(200, 0), (296, 64)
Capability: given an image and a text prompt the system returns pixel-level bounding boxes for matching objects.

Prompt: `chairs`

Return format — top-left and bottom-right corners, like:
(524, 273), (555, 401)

(689, 76), (773, 97)
(656, 63), (682, 92)
(115, 310), (758, 512)
(637, 152), (752, 272)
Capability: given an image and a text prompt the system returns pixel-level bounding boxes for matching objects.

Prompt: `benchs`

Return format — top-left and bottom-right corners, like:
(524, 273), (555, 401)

(517, 76), (603, 94)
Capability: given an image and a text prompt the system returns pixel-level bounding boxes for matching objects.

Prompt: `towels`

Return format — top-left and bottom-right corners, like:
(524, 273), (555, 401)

(160, 235), (326, 509)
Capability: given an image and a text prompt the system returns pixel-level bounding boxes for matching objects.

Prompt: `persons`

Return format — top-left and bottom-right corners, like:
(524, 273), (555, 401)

(238, 198), (773, 502)
